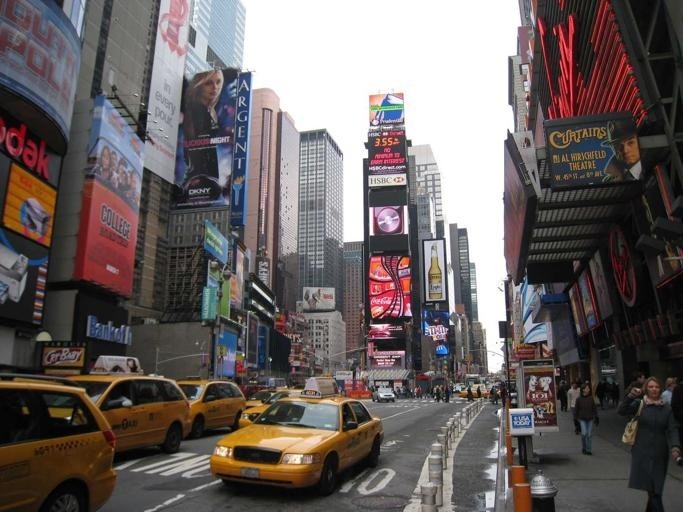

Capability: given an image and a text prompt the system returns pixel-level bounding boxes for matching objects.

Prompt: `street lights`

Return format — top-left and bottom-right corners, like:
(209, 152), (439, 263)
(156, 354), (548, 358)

(206, 254), (233, 381)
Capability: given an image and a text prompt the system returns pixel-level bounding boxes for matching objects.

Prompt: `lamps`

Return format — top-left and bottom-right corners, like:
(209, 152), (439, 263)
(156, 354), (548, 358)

(108, 93), (170, 141)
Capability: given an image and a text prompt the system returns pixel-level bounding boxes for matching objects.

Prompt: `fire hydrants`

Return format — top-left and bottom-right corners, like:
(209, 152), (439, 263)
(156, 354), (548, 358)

(530, 469), (561, 512)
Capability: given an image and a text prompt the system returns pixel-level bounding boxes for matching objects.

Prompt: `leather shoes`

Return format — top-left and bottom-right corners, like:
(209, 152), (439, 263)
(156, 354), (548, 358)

(583, 449), (592, 455)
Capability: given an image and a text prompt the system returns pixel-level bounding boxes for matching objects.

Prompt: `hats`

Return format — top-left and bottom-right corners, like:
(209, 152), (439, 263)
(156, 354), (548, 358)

(601, 121), (636, 145)
(664, 376), (678, 388)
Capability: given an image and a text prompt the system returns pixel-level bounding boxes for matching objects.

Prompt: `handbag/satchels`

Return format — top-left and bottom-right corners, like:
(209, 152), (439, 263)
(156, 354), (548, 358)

(621, 419), (639, 446)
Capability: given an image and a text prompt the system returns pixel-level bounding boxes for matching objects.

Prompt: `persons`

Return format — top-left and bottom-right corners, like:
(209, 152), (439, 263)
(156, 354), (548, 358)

(91, 146), (139, 208)
(395, 384), (517, 407)
(177, 70), (224, 200)
(617, 376), (682, 512)
(558, 373), (683, 467)
(601, 119), (643, 183)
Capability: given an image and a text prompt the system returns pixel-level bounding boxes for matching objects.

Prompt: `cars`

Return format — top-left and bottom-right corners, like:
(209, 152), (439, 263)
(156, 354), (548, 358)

(14, 356), (195, 460)
(201, 377), (386, 497)
(451, 372), (518, 404)
(162, 371), (345, 443)
(0, 368), (122, 512)
(372, 386), (397, 404)
